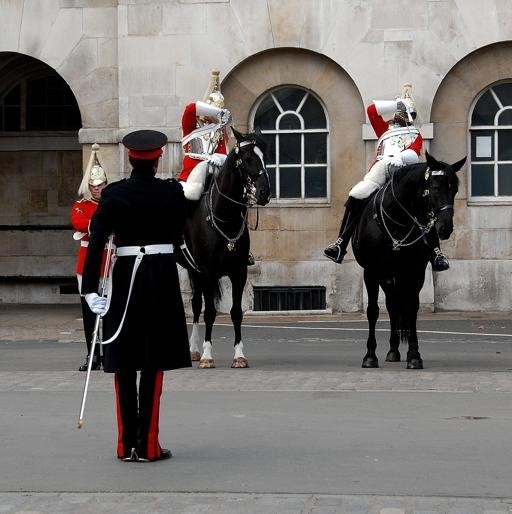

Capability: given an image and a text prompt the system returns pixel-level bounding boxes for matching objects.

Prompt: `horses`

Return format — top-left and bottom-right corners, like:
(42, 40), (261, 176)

(184, 124), (274, 369)
(349, 146), (469, 372)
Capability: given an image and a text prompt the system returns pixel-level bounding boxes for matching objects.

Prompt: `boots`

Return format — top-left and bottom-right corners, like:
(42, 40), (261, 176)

(423, 215), (449, 272)
(325, 208), (354, 265)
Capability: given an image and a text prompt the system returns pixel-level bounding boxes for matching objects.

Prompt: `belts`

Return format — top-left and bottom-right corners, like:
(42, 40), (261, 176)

(116, 245), (175, 256)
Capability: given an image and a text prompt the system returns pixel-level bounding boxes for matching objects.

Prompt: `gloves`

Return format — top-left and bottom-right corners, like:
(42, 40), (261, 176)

(387, 156), (406, 167)
(84, 292), (108, 312)
(218, 109), (233, 124)
(396, 100), (406, 114)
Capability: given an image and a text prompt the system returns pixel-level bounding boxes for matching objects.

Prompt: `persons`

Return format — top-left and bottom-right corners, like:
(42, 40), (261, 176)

(174, 92), (256, 267)
(322, 98), (453, 271)
(81, 130), (199, 464)
(68, 175), (112, 373)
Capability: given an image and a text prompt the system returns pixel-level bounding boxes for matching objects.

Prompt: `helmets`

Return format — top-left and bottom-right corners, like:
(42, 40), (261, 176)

(204, 93), (227, 112)
(87, 166), (106, 187)
(399, 98), (414, 116)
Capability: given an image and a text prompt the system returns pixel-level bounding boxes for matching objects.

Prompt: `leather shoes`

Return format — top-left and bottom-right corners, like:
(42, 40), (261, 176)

(79, 355), (101, 371)
(121, 449), (172, 462)
